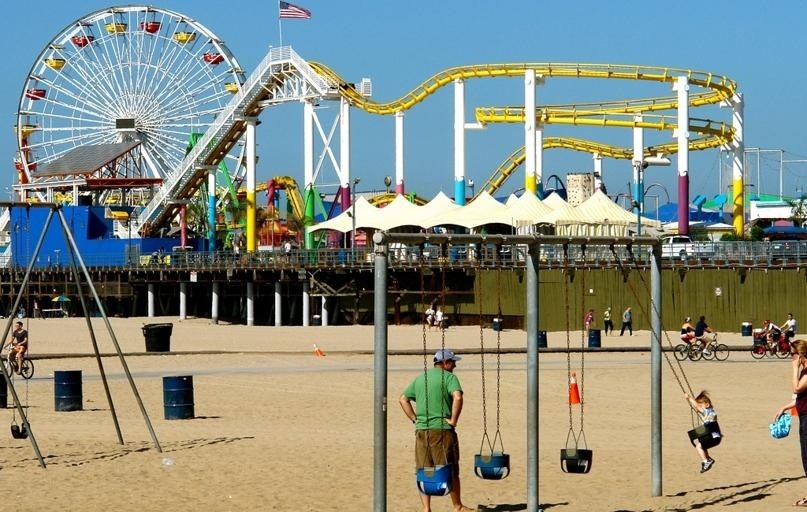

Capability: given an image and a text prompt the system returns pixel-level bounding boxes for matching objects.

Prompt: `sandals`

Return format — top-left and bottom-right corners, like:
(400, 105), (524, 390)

(791, 497), (807, 506)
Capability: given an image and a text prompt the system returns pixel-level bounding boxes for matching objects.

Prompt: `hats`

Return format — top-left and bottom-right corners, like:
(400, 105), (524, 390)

(432, 349), (462, 365)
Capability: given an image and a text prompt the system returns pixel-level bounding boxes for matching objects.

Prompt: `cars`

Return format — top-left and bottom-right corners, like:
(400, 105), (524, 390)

(421, 244), (512, 263)
(765, 240), (805, 255)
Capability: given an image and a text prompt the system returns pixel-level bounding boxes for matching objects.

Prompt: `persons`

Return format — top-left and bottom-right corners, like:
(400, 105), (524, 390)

(584, 309), (593, 334)
(602, 306), (613, 336)
(435, 306), (444, 332)
(619, 305), (635, 336)
(399, 348), (477, 512)
(778, 313), (796, 355)
(33, 298), (38, 310)
(685, 390), (723, 473)
(694, 315), (713, 354)
(158, 246), (167, 264)
(4, 320), (27, 375)
(59, 302), (69, 318)
(772, 339), (806, 505)
(151, 249), (160, 264)
(424, 304), (436, 330)
(284, 240), (292, 262)
(280, 240), (287, 263)
(62, 303), (67, 311)
(757, 320), (783, 357)
(679, 316), (696, 351)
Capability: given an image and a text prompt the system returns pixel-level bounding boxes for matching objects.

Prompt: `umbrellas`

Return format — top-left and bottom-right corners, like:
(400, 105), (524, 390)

(51, 294), (72, 308)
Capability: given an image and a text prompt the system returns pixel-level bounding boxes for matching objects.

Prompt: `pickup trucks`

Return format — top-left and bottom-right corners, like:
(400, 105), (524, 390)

(647, 232), (716, 262)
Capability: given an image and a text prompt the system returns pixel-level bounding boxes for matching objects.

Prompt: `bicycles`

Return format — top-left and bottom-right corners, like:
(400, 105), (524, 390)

(673, 329), (796, 361)
(0, 345), (34, 380)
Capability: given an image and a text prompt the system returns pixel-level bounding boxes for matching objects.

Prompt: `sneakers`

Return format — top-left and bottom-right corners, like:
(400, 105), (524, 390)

(704, 456), (715, 470)
(702, 348), (711, 355)
(699, 462), (712, 474)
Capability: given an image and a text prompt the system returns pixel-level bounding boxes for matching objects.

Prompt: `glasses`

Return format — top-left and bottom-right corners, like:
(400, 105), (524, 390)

(790, 352), (798, 357)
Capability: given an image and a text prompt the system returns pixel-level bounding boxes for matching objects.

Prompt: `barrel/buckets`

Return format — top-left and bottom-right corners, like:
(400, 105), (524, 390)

(54, 369), (83, 411)
(162, 375), (195, 420)
(741, 321), (752, 336)
(0, 371), (8, 406)
(142, 322), (173, 352)
(537, 330), (547, 347)
(493, 318), (503, 331)
(588, 329), (601, 347)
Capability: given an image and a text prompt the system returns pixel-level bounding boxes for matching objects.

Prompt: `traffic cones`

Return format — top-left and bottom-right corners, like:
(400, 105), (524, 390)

(789, 391), (798, 417)
(312, 342), (326, 358)
(566, 371), (583, 405)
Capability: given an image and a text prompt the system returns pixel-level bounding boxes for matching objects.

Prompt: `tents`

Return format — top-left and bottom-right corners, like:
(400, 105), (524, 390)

(513, 186), (662, 262)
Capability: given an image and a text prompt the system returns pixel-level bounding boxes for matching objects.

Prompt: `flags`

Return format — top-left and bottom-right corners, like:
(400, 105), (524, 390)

(279, 1), (313, 20)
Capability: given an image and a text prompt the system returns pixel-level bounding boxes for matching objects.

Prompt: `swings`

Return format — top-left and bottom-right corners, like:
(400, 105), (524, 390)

(414, 239), (454, 496)
(8, 206), (30, 439)
(560, 243), (592, 474)
(474, 241), (510, 480)
(611, 245), (722, 448)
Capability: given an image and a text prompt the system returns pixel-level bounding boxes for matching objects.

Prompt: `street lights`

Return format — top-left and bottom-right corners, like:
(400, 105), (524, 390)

(123, 216), (139, 266)
(727, 183), (756, 224)
(52, 248), (63, 268)
(13, 223), (21, 271)
(350, 176), (361, 268)
